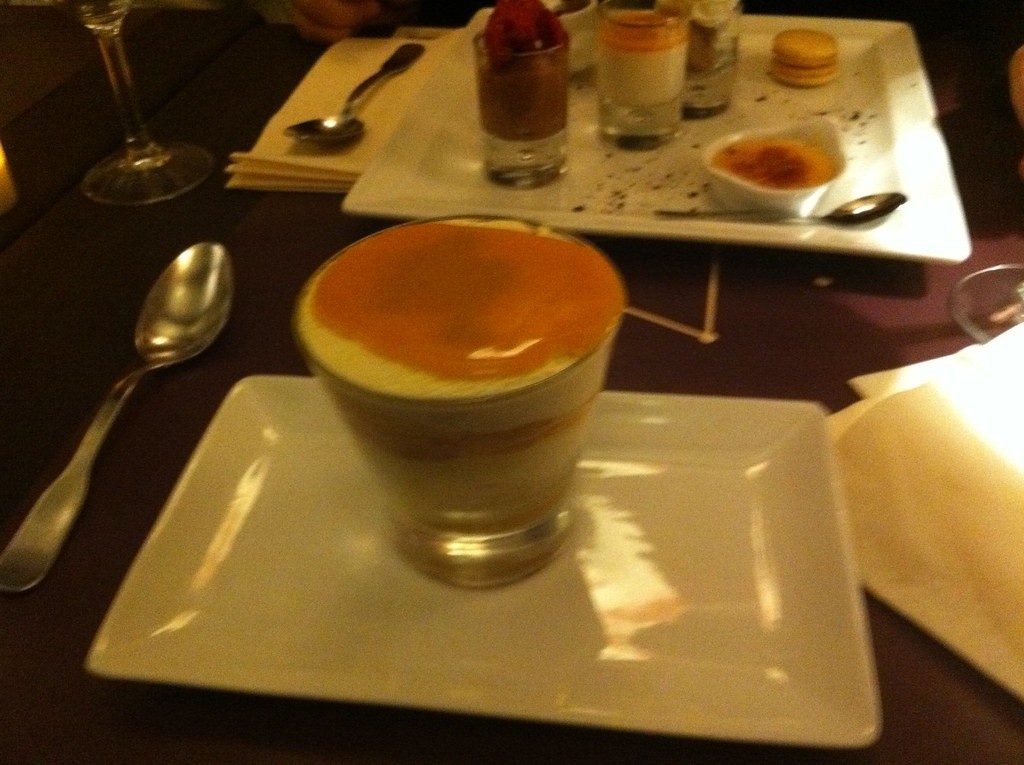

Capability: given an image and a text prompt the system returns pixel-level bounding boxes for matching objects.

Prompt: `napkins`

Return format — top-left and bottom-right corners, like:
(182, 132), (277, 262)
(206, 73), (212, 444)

(222, 37), (431, 193)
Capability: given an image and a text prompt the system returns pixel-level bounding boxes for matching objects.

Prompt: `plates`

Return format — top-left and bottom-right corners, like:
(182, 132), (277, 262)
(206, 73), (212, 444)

(86, 375), (883, 751)
(339, 14), (973, 268)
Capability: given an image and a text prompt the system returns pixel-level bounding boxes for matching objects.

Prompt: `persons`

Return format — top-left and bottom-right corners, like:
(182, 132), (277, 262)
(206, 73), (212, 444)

(292, 0), (1024, 187)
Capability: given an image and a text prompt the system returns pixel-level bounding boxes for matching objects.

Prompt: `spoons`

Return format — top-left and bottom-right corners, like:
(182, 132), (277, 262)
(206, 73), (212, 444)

(654, 192), (909, 221)
(0, 241), (236, 600)
(285, 41), (426, 145)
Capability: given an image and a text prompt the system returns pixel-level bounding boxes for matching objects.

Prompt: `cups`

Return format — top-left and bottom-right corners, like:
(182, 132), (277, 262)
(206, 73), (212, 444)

(540, 0), (605, 77)
(592, 0), (700, 151)
(654, 0), (745, 120)
(293, 212), (628, 590)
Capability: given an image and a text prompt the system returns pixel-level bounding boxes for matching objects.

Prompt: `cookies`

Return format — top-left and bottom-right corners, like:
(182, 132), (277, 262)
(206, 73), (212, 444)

(769, 27), (841, 88)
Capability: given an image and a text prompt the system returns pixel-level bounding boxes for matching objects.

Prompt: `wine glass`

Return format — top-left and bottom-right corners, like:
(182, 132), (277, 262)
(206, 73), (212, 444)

(50, 1), (217, 205)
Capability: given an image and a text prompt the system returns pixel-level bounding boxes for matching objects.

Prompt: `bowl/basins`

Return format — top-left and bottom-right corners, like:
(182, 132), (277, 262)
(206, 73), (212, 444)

(705, 112), (847, 220)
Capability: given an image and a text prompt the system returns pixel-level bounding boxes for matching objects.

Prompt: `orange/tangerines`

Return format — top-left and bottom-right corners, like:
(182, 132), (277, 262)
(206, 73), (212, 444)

(598, 12), (687, 48)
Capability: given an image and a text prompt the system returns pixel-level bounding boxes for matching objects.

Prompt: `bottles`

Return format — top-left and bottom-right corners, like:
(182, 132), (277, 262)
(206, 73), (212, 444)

(470, 32), (573, 192)
